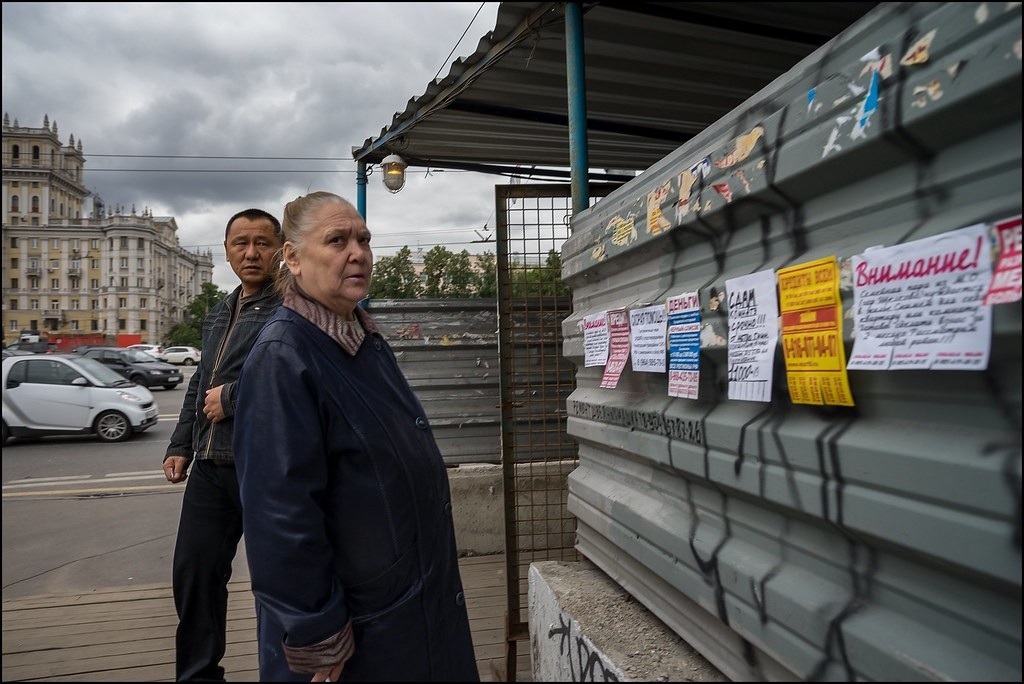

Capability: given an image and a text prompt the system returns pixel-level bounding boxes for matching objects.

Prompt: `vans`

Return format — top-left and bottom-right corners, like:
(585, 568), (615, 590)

(5, 342), (58, 358)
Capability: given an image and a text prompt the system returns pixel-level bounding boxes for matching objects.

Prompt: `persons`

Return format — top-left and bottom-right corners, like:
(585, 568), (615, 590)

(161, 207), (285, 684)
(236, 192), (482, 684)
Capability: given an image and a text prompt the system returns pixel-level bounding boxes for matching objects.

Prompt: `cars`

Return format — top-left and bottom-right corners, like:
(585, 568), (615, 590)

(1, 354), (160, 443)
(2, 350), (35, 363)
(79, 345), (184, 392)
(126, 344), (161, 361)
(160, 346), (202, 365)
